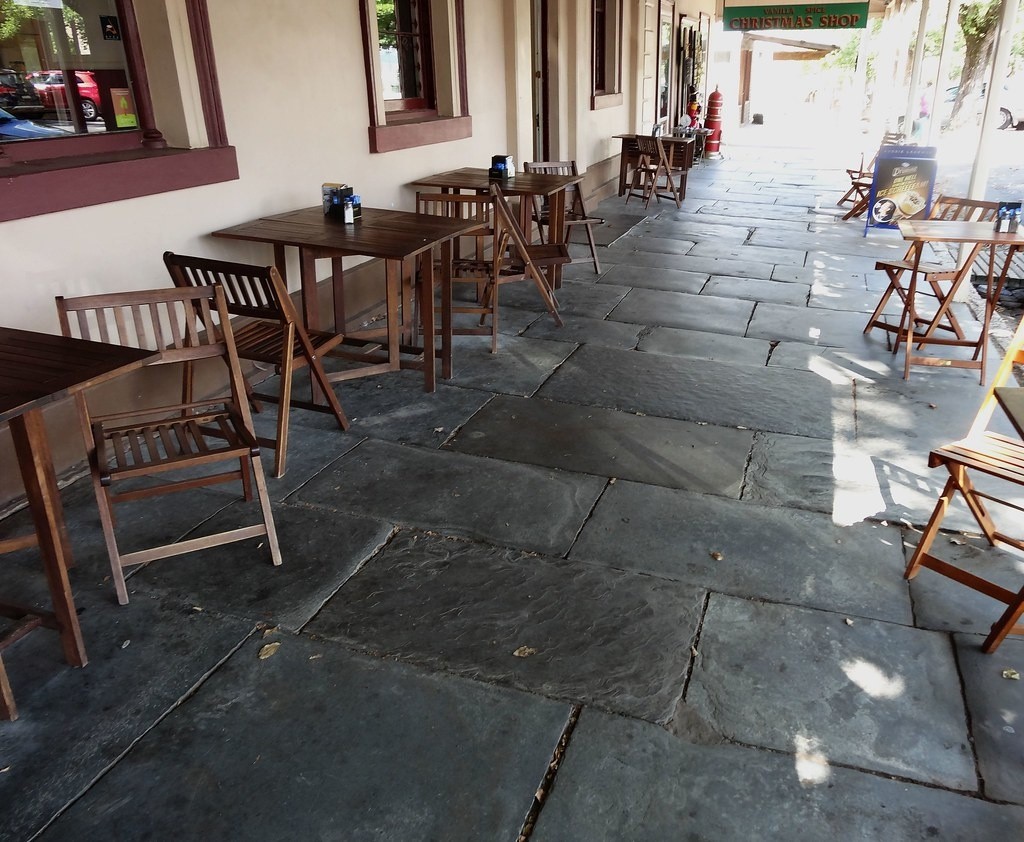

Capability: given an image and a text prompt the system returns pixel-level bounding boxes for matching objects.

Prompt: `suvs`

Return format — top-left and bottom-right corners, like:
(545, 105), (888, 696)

(27, 70), (103, 121)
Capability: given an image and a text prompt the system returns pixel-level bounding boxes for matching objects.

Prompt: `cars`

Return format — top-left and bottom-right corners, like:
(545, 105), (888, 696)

(0, 69), (45, 118)
(896, 52), (1024, 135)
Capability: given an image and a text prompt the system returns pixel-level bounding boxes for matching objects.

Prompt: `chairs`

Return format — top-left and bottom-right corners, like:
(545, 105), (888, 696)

(524, 160), (605, 274)
(162, 250), (351, 479)
(864, 194), (1001, 351)
(837, 132), (919, 220)
(690, 134), (706, 170)
(54, 283), (283, 605)
(626, 135), (681, 209)
(412, 191), (498, 355)
(904, 316), (1024, 655)
(479, 182), (564, 328)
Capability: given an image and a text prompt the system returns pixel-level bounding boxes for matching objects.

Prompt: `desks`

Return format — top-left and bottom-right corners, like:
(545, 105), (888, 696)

(0, 325), (163, 720)
(893, 219), (1024, 386)
(410, 167), (585, 305)
(673, 127), (715, 166)
(612, 134), (695, 201)
(211, 204), (491, 404)
